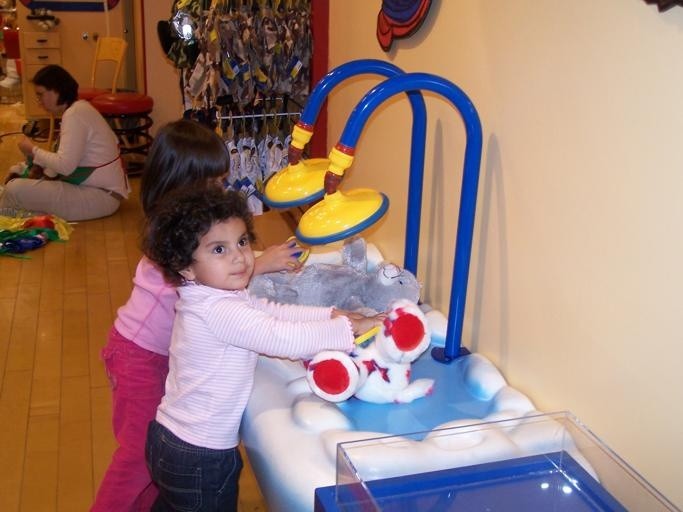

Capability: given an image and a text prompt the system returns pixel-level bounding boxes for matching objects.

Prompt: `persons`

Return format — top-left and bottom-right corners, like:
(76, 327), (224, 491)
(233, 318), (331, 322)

(90, 120), (309, 512)
(138, 184), (388, 512)
(0, 63), (131, 222)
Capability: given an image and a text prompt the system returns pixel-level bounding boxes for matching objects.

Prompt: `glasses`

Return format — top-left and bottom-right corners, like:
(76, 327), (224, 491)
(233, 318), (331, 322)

(32, 89), (50, 105)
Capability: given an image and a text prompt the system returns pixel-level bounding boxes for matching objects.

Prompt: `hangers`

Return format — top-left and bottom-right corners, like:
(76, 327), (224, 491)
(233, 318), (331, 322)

(209, 95), (294, 142)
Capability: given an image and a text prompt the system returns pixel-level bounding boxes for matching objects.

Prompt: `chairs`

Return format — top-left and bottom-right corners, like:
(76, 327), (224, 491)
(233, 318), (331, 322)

(45, 36), (136, 160)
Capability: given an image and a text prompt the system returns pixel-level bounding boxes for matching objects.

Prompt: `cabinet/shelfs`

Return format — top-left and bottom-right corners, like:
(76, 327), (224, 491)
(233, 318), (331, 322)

(19, 32), (62, 119)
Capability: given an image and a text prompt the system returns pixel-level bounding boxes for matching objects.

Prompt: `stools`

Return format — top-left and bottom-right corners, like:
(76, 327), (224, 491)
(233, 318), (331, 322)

(90, 92), (156, 178)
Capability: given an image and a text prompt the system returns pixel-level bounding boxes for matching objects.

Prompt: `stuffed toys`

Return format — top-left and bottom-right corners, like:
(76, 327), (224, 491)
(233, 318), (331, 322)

(247, 235), (421, 318)
(306, 298), (435, 404)
